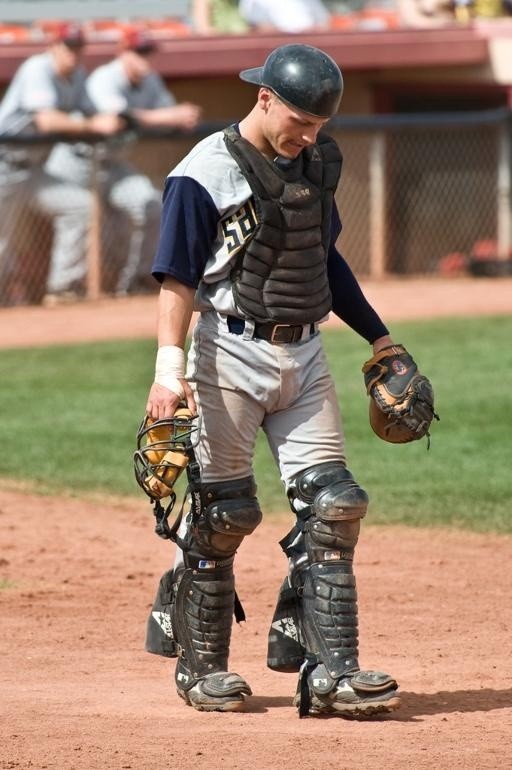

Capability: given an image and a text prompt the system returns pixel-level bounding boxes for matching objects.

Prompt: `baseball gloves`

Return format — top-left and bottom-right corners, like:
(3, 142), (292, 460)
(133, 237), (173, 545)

(363, 344), (434, 442)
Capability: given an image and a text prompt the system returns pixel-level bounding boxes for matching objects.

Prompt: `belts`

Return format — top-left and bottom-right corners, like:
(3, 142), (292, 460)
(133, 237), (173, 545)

(222, 313), (318, 344)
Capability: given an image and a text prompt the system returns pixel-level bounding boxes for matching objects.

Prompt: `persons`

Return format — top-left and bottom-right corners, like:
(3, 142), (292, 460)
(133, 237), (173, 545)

(45, 31), (203, 296)
(0, 20), (128, 308)
(134, 42), (440, 716)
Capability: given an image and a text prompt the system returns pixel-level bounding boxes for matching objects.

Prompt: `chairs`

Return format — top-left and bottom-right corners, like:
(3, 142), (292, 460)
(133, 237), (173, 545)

(1, 10), (396, 49)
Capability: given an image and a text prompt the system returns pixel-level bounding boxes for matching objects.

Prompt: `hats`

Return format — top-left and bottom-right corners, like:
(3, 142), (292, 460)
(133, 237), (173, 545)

(121, 32), (161, 54)
(51, 27), (86, 51)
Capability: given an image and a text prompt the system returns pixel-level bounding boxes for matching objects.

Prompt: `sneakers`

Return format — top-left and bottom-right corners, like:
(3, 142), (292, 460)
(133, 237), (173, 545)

(288, 665), (403, 716)
(172, 668), (245, 711)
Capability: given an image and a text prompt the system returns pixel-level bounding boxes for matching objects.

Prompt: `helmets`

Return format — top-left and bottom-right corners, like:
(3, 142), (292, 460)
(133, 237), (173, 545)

(239, 41), (347, 120)
